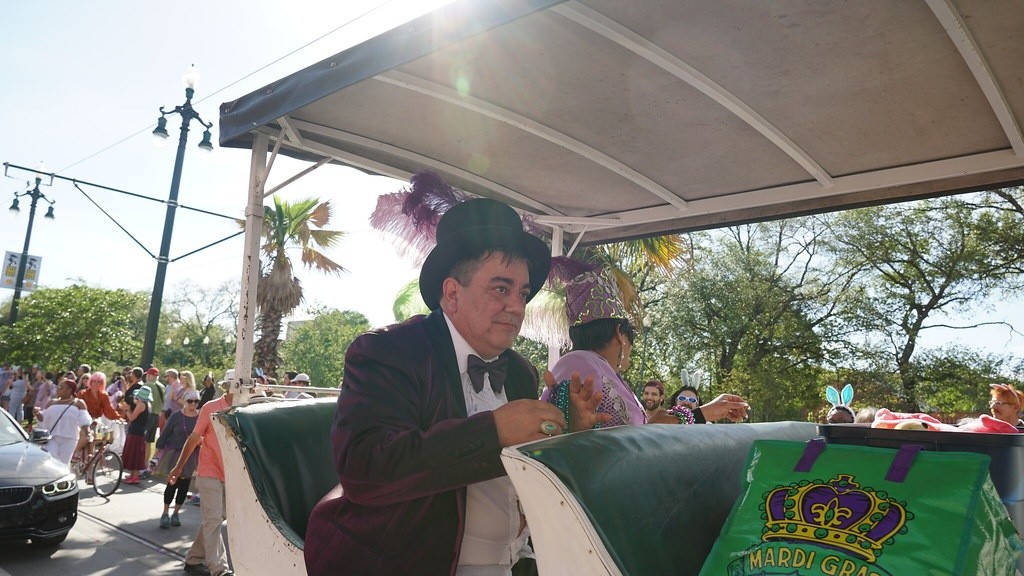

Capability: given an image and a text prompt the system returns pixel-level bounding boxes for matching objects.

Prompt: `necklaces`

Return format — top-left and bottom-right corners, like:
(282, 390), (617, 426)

(548, 378), (600, 434)
(183, 408), (200, 448)
(669, 405), (695, 425)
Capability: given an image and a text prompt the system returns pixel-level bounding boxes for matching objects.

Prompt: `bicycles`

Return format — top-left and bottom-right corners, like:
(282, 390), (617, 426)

(72, 426), (123, 497)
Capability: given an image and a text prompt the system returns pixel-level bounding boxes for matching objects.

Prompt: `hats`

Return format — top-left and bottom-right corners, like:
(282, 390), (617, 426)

(143, 368), (159, 376)
(547, 252), (648, 336)
(419, 198), (551, 311)
(644, 381), (664, 406)
(289, 373), (310, 382)
(137, 385), (154, 402)
(130, 390), (140, 397)
(217, 369), (235, 385)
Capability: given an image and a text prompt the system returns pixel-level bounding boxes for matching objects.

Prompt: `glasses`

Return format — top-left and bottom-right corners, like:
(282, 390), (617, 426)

(186, 399), (200, 404)
(675, 396), (698, 404)
(989, 400), (1017, 405)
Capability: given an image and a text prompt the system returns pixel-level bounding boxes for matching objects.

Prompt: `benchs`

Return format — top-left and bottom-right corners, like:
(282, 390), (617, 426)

(500, 420), (820, 576)
(212, 394), (339, 576)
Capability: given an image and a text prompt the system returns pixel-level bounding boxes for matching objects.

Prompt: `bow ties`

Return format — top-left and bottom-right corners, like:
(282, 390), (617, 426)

(467, 354), (508, 393)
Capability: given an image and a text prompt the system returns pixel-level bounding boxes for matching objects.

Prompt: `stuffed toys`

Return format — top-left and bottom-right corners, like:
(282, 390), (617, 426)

(871, 408), (1019, 433)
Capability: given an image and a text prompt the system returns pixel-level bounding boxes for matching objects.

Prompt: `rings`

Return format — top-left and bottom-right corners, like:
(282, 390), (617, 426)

(540, 420), (558, 435)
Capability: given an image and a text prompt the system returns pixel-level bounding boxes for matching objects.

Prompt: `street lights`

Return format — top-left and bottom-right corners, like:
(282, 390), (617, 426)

(139, 76), (216, 372)
(8, 163), (56, 327)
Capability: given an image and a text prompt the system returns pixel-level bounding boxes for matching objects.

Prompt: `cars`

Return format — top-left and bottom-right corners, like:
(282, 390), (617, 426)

(1, 405), (79, 547)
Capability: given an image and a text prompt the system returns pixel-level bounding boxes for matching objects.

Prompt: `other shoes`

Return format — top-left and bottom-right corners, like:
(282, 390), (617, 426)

(184, 563), (209, 576)
(123, 475), (139, 483)
(193, 497), (200, 504)
(139, 471), (146, 479)
(160, 516), (169, 528)
(171, 514), (181, 526)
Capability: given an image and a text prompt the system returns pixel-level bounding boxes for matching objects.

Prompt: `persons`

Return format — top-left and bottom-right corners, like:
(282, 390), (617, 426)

(158, 368), (216, 528)
(667, 368), (715, 425)
(255, 367), (317, 398)
(988, 383), (1024, 429)
(0, 362), (166, 484)
(304, 197), (612, 576)
(642, 379), (665, 418)
(826, 385), (855, 423)
(537, 269), (681, 428)
(167, 368), (238, 576)
